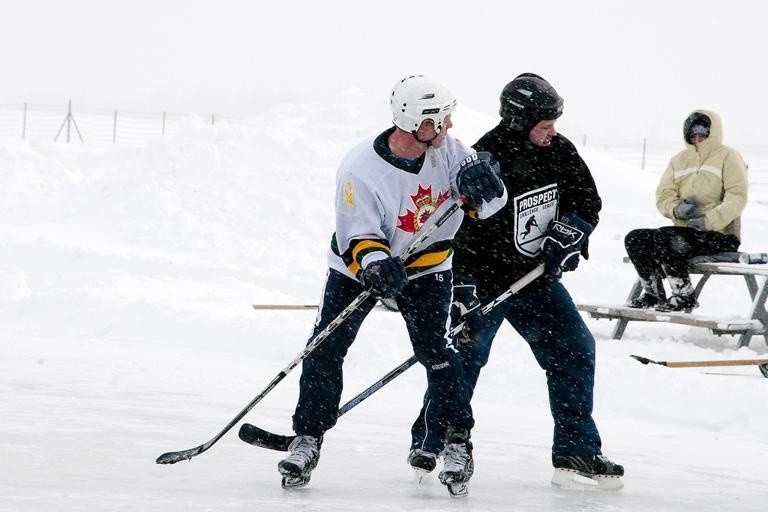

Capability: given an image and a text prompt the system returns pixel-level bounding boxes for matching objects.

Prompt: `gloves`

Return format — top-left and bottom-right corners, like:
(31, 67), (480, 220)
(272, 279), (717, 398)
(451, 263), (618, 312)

(457, 153), (504, 205)
(540, 212), (592, 280)
(450, 285), (483, 340)
(674, 202), (696, 219)
(688, 214), (704, 231)
(360, 256), (408, 300)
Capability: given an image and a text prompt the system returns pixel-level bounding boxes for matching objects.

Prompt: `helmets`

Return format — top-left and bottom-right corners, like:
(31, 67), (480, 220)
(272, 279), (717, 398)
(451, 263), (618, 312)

(500, 73), (563, 130)
(391, 74), (457, 134)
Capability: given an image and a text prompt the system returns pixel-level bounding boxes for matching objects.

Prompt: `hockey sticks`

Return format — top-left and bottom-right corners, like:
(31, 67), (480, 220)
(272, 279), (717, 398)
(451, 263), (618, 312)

(155, 161), (499, 465)
(239, 262), (548, 452)
(630, 354), (768, 367)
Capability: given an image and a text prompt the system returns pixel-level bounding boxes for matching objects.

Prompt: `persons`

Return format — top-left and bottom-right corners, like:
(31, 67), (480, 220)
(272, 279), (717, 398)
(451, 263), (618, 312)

(625, 108), (748, 313)
(280, 72), (508, 484)
(438, 72), (625, 483)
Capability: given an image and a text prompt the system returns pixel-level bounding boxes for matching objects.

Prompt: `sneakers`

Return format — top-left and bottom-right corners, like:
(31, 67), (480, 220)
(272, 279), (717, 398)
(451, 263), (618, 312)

(409, 449), (436, 471)
(655, 295), (697, 312)
(439, 443), (474, 483)
(279, 433), (323, 477)
(628, 293), (666, 308)
(552, 452), (624, 475)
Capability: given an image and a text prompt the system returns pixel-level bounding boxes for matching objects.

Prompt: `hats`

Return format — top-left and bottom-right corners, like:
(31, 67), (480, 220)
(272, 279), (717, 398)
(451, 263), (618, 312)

(688, 118), (710, 136)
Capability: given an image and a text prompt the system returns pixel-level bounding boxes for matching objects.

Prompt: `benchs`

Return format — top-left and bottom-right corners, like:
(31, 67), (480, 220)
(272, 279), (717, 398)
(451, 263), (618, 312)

(574, 298), (766, 351)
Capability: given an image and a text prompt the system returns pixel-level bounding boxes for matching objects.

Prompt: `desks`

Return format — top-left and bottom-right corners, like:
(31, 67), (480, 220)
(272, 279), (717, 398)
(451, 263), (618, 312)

(611, 252), (768, 357)
